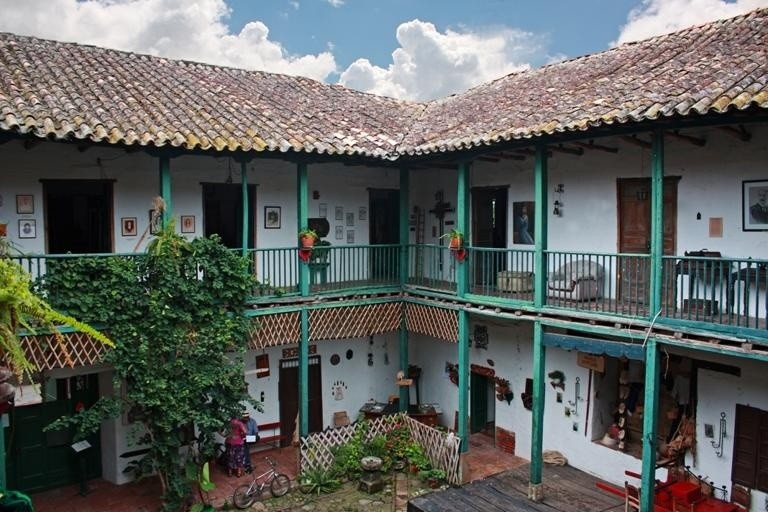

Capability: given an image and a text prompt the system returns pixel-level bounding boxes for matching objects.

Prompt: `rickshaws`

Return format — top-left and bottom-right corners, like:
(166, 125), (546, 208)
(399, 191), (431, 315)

(593, 471), (747, 512)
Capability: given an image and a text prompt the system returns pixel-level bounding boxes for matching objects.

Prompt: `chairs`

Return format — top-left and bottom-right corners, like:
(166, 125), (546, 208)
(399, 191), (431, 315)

(625, 480), (752, 512)
(388, 394), (400, 405)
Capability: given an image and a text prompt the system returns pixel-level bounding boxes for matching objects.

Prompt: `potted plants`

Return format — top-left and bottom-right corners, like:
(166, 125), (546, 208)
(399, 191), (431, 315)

(547, 370), (565, 384)
(491, 376), (513, 406)
(298, 229), (319, 251)
(437, 227), (464, 251)
(443, 361), (459, 387)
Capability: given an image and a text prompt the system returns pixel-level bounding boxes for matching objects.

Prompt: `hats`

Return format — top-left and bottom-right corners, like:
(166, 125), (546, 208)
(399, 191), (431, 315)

(241, 409), (251, 418)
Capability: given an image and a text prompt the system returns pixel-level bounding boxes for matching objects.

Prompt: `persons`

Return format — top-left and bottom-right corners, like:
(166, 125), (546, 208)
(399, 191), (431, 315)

(514, 205), (535, 244)
(240, 409), (259, 474)
(218, 412), (247, 477)
(749, 187), (768, 224)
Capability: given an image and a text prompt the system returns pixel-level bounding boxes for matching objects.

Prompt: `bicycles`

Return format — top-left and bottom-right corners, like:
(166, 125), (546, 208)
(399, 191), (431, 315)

(232, 455), (290, 510)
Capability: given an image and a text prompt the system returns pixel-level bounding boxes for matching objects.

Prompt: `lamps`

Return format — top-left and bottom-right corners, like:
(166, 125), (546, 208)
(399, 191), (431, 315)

(552, 186), (561, 216)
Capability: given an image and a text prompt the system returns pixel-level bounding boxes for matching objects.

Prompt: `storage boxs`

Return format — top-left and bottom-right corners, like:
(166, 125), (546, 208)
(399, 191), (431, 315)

(682, 298), (719, 317)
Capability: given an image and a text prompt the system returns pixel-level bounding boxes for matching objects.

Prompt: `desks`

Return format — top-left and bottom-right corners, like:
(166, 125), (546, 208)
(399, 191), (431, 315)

(730, 267), (768, 319)
(359, 401), (444, 429)
(676, 251), (728, 314)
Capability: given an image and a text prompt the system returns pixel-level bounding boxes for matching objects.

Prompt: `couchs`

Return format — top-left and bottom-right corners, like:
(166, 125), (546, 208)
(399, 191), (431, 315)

(545, 259), (603, 302)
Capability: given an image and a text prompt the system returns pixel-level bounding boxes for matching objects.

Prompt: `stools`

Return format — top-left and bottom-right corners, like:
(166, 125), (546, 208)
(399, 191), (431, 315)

(496, 270), (534, 293)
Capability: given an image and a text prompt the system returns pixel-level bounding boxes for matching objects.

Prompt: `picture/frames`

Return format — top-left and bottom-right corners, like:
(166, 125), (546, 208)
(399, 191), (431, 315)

(15, 194), (36, 238)
(741, 179), (768, 232)
(708, 217), (723, 239)
(263, 205), (282, 229)
(317, 203), (368, 243)
(121, 209), (196, 237)
(513, 201), (535, 245)
